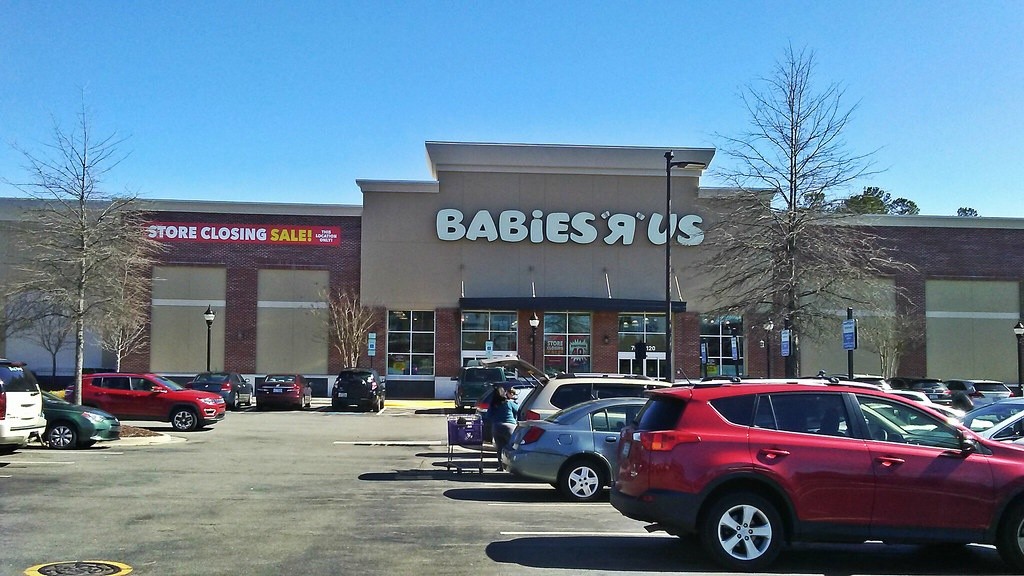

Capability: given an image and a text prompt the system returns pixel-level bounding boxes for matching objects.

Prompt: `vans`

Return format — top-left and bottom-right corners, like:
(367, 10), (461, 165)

(451, 365), (508, 411)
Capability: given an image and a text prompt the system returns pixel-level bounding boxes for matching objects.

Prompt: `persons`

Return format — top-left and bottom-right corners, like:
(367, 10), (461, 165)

(488, 385), (519, 470)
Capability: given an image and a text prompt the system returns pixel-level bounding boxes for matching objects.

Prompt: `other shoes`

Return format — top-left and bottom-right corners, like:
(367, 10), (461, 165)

(497, 466), (504, 471)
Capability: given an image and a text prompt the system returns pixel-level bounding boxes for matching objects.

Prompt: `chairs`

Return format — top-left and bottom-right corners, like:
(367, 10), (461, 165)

(788, 402), (840, 437)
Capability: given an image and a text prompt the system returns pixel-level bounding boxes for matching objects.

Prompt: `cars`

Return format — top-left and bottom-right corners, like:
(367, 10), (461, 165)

(501, 397), (649, 503)
(184, 371), (253, 411)
(39, 390), (122, 450)
(256, 374), (313, 410)
(695, 370), (1024, 449)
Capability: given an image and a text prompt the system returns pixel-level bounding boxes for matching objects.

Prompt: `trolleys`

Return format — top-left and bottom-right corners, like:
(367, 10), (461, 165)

(447, 412), (487, 477)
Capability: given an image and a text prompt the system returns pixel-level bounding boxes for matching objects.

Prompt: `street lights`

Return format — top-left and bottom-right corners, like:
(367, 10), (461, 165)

(527, 311), (541, 367)
(1013, 321), (1024, 389)
(662, 149), (707, 379)
(202, 303), (217, 372)
(763, 318), (776, 381)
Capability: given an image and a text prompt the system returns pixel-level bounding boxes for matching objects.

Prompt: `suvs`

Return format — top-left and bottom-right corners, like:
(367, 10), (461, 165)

(332, 367), (387, 413)
(479, 355), (677, 429)
(609, 371), (1024, 572)
(0, 357), (48, 454)
(476, 381), (541, 444)
(63, 372), (225, 432)
